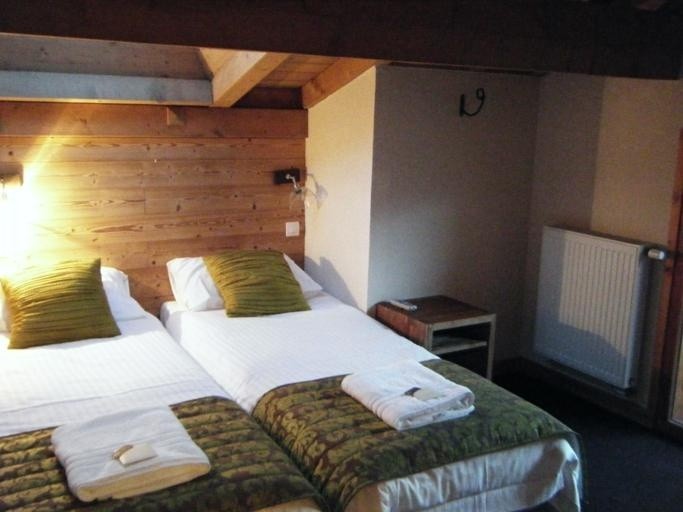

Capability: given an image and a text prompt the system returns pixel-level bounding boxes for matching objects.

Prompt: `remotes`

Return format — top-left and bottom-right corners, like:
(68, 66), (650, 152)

(390, 299), (418, 311)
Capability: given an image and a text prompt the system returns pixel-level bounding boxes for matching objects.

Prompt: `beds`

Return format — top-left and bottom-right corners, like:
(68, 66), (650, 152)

(0, 310), (325, 511)
(159, 290), (575, 512)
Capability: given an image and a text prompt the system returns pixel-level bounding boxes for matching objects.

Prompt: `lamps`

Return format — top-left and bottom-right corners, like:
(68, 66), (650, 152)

(271, 168), (318, 212)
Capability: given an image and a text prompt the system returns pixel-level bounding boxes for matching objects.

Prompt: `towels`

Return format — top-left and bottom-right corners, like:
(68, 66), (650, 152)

(50, 399), (212, 504)
(340, 359), (477, 433)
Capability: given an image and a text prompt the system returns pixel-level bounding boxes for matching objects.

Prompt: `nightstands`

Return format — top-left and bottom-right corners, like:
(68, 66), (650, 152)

(376, 292), (497, 382)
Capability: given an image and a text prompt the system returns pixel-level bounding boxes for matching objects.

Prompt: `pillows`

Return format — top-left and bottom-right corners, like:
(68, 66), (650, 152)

(0, 264), (146, 334)
(201, 249), (312, 318)
(165, 250), (324, 313)
(0, 257), (122, 349)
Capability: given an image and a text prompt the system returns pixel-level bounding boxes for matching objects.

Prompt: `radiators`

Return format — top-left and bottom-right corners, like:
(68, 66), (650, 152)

(533, 224), (651, 398)
